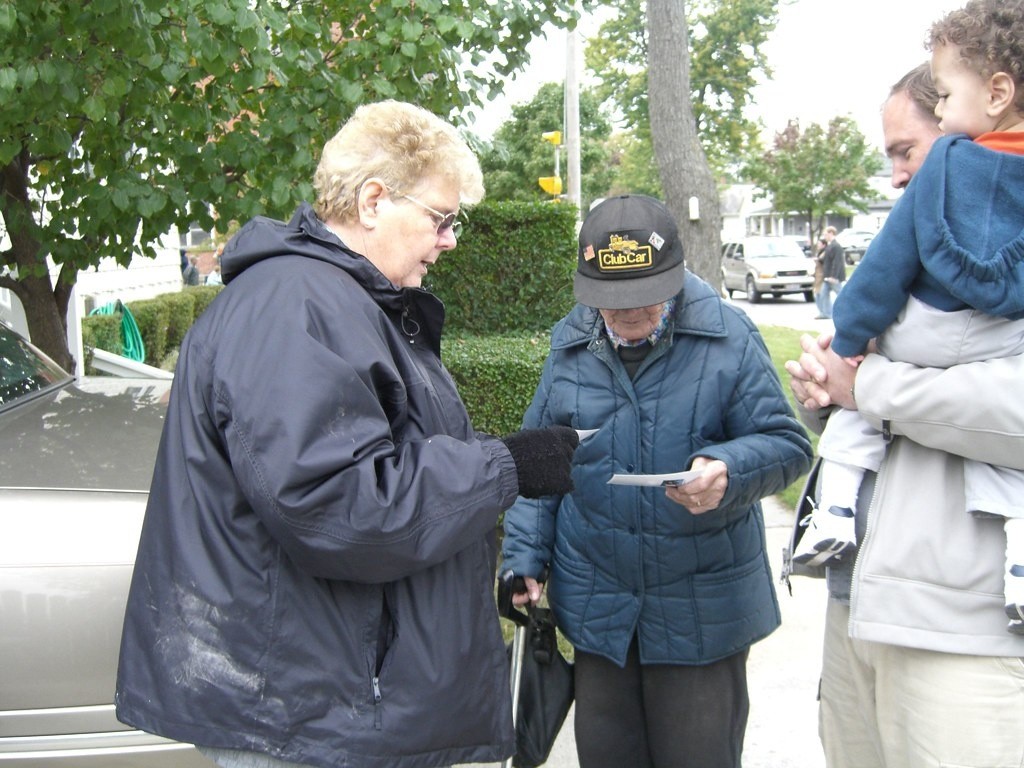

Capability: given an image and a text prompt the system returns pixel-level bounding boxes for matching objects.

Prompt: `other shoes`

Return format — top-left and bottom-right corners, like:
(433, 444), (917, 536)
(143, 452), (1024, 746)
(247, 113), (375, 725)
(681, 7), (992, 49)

(815, 314), (830, 318)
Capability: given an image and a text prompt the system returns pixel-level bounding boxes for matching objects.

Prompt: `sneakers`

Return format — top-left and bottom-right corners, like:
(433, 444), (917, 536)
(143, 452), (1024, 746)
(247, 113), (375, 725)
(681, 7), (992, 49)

(791, 494), (859, 570)
(1002, 564), (1024, 635)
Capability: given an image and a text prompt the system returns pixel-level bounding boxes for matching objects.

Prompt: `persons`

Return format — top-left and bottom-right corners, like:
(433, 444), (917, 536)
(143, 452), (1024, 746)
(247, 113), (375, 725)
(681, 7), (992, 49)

(783, 0), (1024, 638)
(494, 195), (814, 768)
(112, 99), (578, 768)
(781, 61), (1024, 768)
(814, 224), (846, 319)
(180, 244), (226, 286)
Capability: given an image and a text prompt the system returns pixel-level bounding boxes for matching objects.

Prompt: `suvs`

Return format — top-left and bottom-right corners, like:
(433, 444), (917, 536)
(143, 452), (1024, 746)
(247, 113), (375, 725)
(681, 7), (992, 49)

(724, 239), (818, 307)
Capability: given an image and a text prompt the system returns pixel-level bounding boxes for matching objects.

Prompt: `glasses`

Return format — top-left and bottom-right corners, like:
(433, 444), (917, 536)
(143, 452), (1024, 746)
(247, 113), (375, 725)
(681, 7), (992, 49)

(385, 185), (463, 239)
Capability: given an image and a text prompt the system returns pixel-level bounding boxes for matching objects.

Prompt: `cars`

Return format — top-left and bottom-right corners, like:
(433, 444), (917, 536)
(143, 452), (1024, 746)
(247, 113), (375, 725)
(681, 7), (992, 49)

(2, 322), (248, 768)
(725, 227), (879, 283)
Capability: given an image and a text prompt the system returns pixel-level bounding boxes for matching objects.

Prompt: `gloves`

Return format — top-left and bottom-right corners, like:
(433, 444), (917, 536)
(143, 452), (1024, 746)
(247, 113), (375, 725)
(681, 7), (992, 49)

(501, 425), (581, 499)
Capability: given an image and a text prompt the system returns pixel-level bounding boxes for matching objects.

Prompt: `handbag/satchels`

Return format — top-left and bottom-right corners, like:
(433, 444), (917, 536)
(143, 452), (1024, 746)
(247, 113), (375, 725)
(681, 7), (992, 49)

(497, 567), (576, 768)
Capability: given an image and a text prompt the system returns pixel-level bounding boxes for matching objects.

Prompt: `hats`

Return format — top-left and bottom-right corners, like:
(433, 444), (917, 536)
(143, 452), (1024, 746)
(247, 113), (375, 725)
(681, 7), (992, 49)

(573, 194), (685, 309)
(190, 255), (199, 261)
(213, 266), (220, 271)
(217, 248), (223, 256)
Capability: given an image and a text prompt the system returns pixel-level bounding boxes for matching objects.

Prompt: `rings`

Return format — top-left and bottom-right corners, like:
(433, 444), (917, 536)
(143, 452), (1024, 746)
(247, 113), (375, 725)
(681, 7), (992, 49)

(697, 501), (701, 507)
